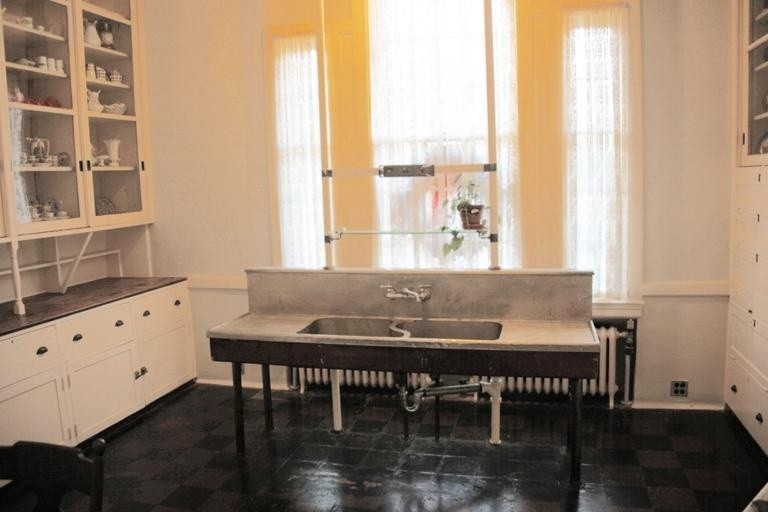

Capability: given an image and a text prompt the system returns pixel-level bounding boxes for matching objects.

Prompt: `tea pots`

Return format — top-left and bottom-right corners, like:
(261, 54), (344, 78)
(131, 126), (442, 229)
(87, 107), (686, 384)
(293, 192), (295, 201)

(26, 136), (50, 163)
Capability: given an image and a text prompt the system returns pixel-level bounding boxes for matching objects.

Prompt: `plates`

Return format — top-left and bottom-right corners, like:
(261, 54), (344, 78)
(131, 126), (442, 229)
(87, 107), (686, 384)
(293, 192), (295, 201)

(756, 132), (768, 153)
(94, 197), (117, 216)
(27, 204), (70, 222)
(13, 57), (36, 67)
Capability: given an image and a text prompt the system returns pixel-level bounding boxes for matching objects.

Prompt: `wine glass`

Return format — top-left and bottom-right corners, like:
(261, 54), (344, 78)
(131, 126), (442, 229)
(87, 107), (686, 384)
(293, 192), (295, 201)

(93, 139), (121, 167)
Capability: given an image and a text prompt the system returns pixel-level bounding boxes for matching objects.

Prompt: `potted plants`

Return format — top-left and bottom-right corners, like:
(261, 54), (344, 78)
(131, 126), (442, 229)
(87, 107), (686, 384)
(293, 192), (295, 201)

(437, 172), (495, 255)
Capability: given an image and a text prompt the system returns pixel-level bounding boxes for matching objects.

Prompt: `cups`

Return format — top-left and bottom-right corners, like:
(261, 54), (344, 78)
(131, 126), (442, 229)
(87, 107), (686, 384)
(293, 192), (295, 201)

(19, 152), (58, 167)
(15, 16), (44, 30)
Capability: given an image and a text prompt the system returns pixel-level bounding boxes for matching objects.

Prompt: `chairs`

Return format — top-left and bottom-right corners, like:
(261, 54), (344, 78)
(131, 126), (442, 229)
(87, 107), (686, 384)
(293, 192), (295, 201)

(0, 438), (106, 512)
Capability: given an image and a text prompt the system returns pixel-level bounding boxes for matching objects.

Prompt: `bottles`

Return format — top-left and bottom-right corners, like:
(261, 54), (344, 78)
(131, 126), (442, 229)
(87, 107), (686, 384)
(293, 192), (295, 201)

(86, 88), (104, 113)
(37, 56), (64, 73)
(84, 18), (123, 83)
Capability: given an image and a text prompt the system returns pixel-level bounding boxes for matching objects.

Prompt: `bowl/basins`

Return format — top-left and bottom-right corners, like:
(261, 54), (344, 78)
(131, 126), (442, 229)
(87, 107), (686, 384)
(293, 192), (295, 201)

(104, 103), (127, 114)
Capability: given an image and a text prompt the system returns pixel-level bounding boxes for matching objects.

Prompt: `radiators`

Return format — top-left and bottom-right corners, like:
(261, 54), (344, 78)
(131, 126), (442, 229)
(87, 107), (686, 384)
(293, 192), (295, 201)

(291, 318), (636, 411)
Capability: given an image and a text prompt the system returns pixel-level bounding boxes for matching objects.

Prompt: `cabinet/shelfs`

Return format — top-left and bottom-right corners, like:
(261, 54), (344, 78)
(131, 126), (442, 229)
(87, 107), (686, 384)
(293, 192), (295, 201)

(0, 0), (156, 244)
(723, 1), (768, 462)
(59, 279), (199, 445)
(0, 320), (74, 490)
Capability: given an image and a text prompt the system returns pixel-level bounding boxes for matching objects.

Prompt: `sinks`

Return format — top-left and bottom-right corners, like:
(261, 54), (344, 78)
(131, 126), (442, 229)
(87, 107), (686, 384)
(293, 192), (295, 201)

(293, 314), (411, 339)
(389, 318), (504, 341)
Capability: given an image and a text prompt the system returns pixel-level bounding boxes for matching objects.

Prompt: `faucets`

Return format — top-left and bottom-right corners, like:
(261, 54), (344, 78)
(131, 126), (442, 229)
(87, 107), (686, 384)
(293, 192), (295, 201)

(380, 283), (432, 303)
